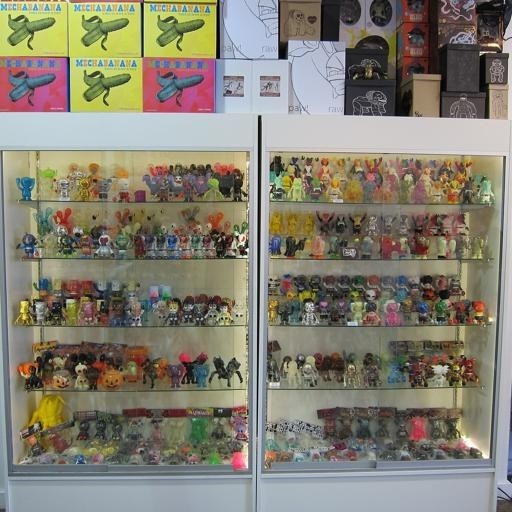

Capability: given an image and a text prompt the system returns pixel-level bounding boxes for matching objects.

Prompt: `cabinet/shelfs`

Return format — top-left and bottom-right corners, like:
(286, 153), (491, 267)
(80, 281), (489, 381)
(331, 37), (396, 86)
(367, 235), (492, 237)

(0, 111), (257, 512)
(257, 112), (512, 512)
